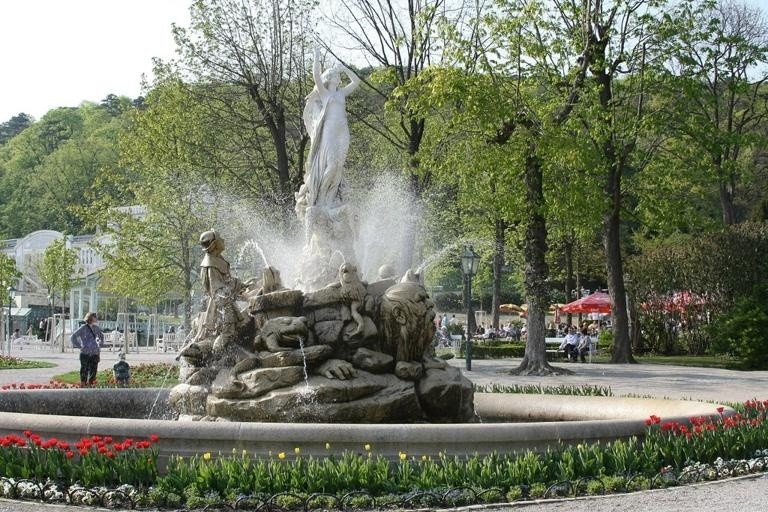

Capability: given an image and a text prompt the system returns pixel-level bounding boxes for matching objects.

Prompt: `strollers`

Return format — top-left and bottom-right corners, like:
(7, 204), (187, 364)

(436, 326), (451, 349)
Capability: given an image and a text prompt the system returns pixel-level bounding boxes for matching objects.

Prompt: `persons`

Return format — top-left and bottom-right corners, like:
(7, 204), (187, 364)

(113, 352), (131, 388)
(25, 325), (33, 336)
(70, 312), (104, 388)
(302, 41), (359, 205)
(435, 312), (613, 363)
(38, 317), (46, 338)
(380, 281), (437, 362)
(200, 227), (255, 341)
(11, 329), (19, 340)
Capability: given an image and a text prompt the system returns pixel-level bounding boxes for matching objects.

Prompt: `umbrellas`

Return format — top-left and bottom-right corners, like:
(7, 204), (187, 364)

(498, 291), (713, 329)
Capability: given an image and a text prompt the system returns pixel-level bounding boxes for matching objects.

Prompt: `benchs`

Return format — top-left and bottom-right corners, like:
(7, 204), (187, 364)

(543, 337), (598, 362)
(155, 331), (185, 353)
(95, 331), (135, 353)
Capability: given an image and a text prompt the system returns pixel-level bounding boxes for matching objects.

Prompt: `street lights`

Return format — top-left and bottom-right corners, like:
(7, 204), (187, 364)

(460, 245), (482, 370)
(7, 285), (17, 356)
